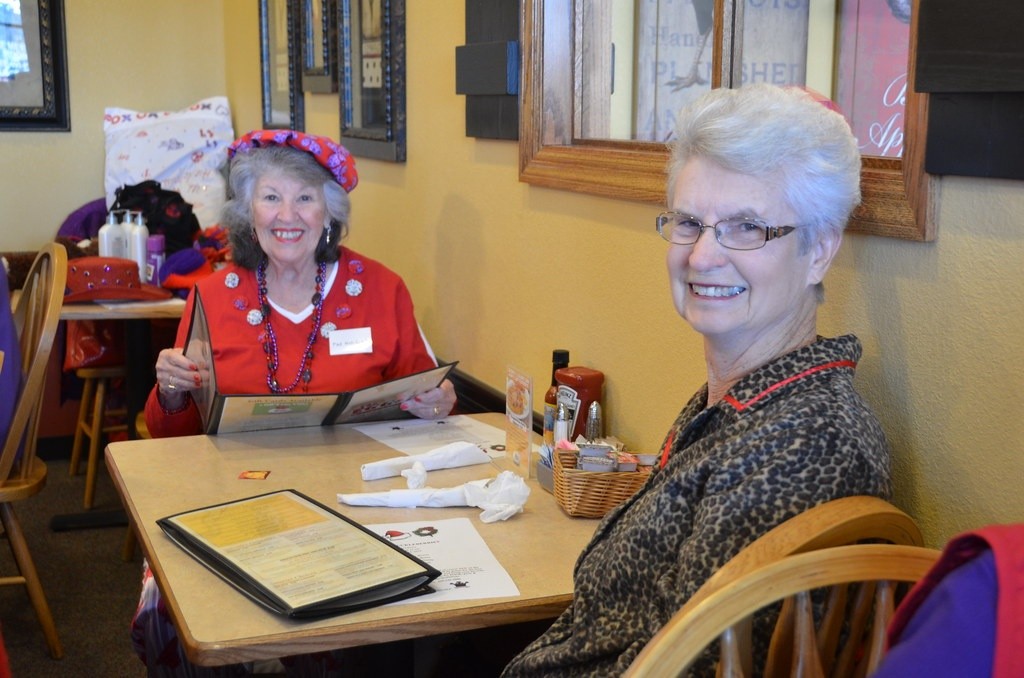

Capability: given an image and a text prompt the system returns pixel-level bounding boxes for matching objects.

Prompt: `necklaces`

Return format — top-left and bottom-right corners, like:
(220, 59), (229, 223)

(257, 257), (327, 393)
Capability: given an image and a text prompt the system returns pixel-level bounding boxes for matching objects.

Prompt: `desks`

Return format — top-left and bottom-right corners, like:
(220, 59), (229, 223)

(58, 296), (188, 442)
(106, 412), (601, 678)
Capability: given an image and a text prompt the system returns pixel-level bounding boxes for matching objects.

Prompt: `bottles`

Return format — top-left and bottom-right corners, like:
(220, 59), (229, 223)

(552, 402), (571, 445)
(542, 349), (570, 446)
(586, 401), (602, 440)
(146, 234), (167, 288)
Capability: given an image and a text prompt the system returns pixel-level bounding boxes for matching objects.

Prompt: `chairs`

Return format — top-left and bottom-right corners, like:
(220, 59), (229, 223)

(0, 244), (947, 677)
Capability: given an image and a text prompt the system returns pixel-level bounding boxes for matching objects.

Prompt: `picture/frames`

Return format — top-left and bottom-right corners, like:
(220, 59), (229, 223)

(259, 0), (408, 162)
(0, 0), (72, 132)
(518, 0), (940, 241)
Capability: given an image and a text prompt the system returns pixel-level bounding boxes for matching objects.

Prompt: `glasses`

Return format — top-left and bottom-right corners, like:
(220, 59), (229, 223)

(656, 210), (809, 250)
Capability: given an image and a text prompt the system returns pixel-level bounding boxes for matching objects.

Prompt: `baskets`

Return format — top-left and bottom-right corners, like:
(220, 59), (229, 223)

(553, 450), (651, 518)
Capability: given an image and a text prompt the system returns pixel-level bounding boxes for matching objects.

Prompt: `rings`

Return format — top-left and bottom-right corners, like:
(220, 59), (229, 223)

(168, 376), (176, 392)
(435, 408), (441, 416)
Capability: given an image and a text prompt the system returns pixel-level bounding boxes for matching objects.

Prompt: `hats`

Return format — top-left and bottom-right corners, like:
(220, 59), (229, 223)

(228, 129), (357, 193)
(63, 256), (173, 304)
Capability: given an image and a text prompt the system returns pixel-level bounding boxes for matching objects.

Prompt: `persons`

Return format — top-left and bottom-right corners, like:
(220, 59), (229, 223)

(497, 83), (908, 678)
(131, 127), (456, 678)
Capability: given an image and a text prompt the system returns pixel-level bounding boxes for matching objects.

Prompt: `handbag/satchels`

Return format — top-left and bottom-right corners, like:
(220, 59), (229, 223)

(63, 318), (128, 371)
(109, 180), (200, 256)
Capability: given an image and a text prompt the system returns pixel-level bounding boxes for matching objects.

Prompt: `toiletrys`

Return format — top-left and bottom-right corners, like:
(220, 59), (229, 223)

(98, 209), (149, 284)
